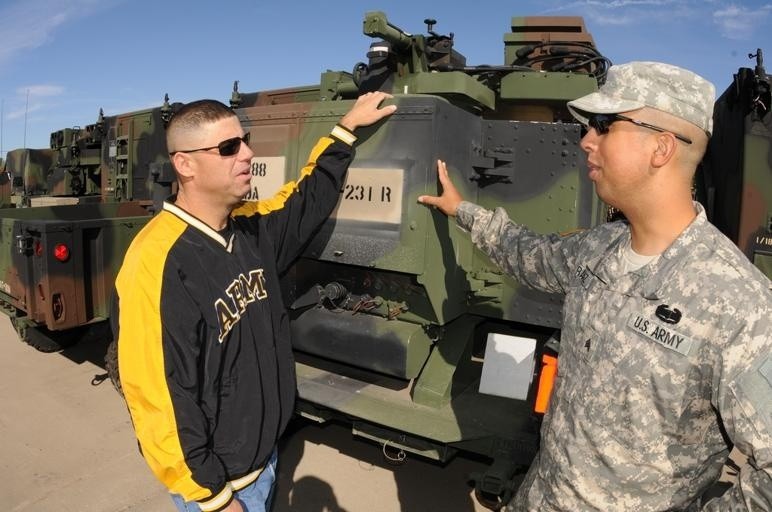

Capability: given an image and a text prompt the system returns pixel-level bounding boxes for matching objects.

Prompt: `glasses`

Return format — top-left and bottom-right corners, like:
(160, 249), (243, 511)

(588, 113), (693, 144)
(169, 131), (251, 157)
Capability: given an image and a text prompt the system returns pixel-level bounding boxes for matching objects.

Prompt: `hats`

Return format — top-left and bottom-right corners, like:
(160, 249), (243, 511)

(566, 61), (716, 139)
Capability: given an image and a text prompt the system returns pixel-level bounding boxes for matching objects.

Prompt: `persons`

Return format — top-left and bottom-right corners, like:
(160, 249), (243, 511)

(112, 87), (398, 512)
(416, 60), (772, 511)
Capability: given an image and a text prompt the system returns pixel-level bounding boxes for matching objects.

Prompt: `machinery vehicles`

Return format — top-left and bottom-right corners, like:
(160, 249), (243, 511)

(0, 10), (771, 506)
